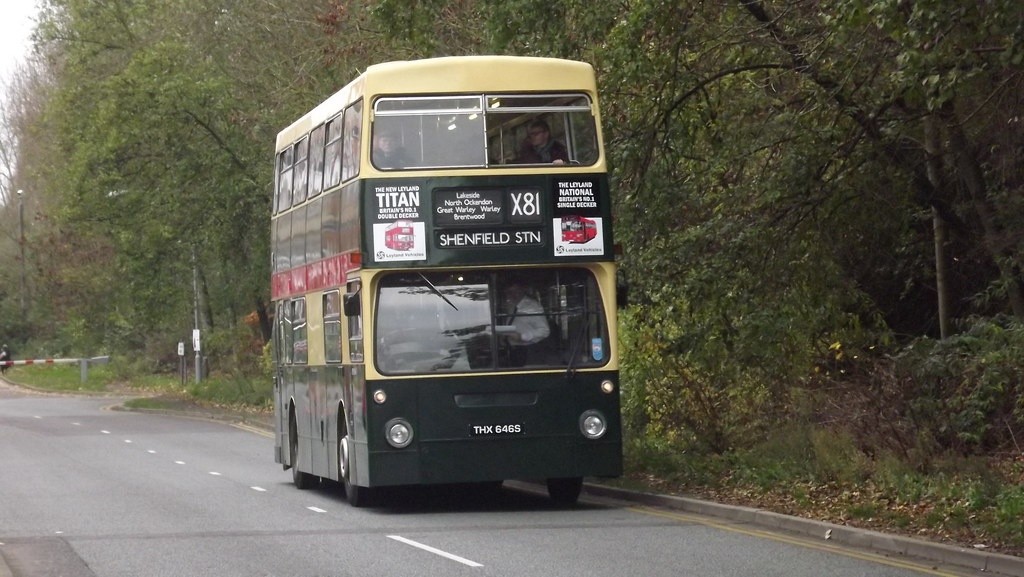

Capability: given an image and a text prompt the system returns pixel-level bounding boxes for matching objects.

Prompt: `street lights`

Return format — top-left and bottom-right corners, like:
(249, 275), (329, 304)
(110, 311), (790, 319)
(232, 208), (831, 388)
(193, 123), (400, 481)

(16, 190), (29, 348)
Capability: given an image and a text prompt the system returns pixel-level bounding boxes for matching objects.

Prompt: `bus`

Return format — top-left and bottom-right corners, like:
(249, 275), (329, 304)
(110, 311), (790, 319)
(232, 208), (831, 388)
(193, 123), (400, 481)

(268, 56), (642, 508)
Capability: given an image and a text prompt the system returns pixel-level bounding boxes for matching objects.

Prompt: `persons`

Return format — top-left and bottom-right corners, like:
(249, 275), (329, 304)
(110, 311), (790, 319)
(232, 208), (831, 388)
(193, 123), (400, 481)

(519, 118), (570, 166)
(496, 275), (554, 368)
(0, 343), (11, 374)
(372, 126), (413, 170)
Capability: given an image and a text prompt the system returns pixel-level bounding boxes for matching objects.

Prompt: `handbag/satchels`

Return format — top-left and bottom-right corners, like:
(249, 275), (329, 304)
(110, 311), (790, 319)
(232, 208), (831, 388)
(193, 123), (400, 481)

(0, 350), (6, 359)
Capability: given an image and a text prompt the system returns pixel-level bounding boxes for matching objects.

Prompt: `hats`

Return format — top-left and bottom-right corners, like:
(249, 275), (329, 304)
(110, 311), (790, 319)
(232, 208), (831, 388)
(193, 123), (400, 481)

(3, 344), (7, 348)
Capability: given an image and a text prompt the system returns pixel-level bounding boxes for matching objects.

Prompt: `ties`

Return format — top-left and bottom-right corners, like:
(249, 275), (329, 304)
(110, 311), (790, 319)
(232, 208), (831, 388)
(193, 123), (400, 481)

(508, 306), (517, 326)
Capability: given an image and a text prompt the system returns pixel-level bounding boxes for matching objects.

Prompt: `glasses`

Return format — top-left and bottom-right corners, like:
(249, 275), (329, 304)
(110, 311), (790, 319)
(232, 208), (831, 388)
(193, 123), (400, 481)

(527, 130), (544, 140)
